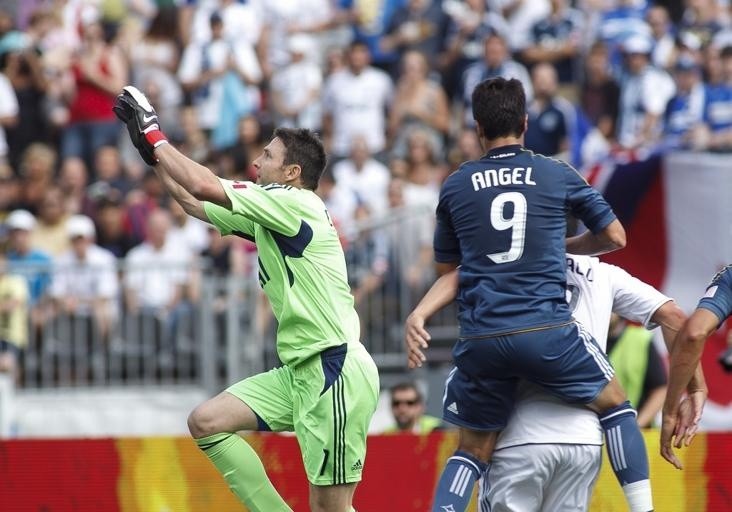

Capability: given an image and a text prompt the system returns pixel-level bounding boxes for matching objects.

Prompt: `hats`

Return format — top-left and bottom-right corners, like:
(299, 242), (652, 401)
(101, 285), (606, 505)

(284, 34), (311, 54)
(621, 36), (652, 55)
(6, 210), (35, 233)
(65, 214), (97, 239)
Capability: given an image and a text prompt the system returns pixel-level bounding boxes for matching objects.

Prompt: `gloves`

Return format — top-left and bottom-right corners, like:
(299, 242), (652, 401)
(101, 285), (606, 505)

(111, 84), (167, 166)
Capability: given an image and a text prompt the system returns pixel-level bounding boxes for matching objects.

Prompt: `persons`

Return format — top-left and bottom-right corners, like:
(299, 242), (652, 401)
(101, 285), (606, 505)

(2, 2), (731, 378)
(107, 81), (381, 511)
(427, 73), (658, 511)
(657, 261), (731, 468)
(385, 384), (447, 433)
(405, 248), (710, 510)
(602, 311), (666, 432)
